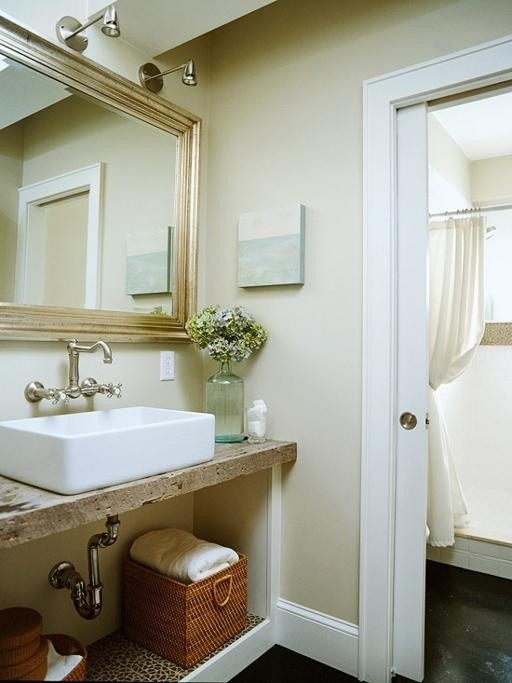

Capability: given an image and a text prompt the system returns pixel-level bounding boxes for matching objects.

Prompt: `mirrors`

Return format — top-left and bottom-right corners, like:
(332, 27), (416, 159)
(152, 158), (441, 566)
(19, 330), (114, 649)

(0, 10), (205, 349)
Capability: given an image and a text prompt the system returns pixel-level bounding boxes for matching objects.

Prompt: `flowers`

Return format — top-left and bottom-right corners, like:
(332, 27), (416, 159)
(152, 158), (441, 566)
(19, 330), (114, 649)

(184, 302), (268, 393)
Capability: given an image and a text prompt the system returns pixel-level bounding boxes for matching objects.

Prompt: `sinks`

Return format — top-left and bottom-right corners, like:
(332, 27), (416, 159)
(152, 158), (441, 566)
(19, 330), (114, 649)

(0, 407), (217, 495)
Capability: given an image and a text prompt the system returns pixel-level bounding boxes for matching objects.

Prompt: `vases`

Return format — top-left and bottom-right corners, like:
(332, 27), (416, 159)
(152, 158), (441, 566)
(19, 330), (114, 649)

(203, 357), (246, 445)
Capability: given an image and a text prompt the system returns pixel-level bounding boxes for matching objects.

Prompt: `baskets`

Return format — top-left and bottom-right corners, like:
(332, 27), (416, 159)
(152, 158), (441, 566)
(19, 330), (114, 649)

(120, 552), (249, 670)
(0, 606), (49, 682)
(40, 632), (89, 682)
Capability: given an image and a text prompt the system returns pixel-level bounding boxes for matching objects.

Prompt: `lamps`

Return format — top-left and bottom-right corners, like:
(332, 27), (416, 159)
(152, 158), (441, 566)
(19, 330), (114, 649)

(57, 5), (201, 94)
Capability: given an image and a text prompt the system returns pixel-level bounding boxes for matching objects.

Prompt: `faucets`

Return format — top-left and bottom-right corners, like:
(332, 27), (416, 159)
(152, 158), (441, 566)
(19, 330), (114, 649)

(67, 338), (113, 399)
